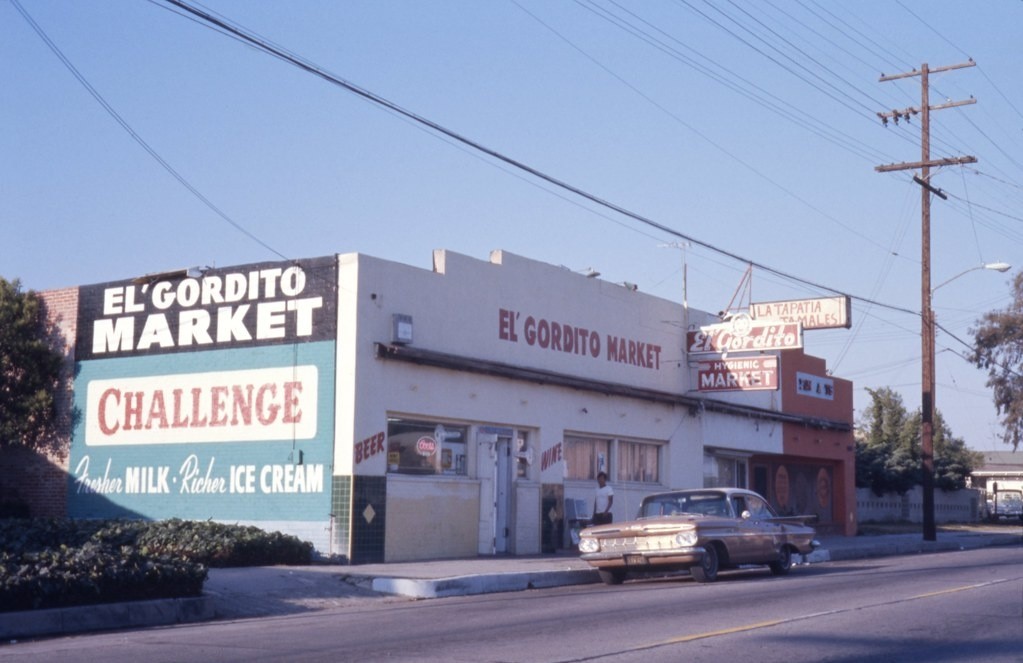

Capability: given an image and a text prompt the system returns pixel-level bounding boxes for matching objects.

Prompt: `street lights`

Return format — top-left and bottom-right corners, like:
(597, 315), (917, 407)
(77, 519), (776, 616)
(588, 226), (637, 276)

(921, 262), (1012, 537)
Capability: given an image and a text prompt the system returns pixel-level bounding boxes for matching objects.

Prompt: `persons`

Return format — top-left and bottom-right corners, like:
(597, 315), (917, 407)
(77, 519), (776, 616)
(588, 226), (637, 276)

(592, 471), (614, 525)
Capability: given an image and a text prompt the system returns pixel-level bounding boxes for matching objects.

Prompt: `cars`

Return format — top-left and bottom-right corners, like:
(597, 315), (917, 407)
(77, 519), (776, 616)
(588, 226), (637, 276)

(578, 487), (820, 584)
